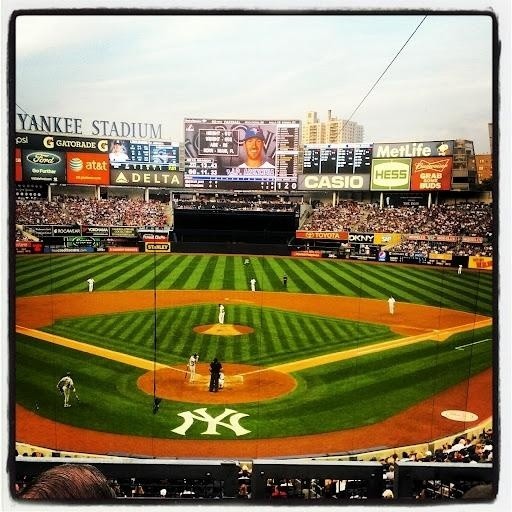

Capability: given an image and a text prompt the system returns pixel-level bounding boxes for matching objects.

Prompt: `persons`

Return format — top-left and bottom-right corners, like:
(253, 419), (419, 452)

(458, 263), (464, 273)
(250, 277), (256, 292)
(283, 275), (287, 287)
(218, 303), (225, 324)
(187, 352), (200, 382)
(209, 358), (223, 392)
(15, 196), (168, 242)
(108, 140), (129, 161)
(216, 372), (225, 389)
(86, 276), (94, 292)
(388, 294), (396, 314)
(301, 200), (493, 256)
(229, 127), (276, 176)
(56, 372), (77, 408)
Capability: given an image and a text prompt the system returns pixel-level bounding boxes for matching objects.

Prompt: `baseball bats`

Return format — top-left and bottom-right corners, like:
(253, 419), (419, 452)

(185, 365), (189, 379)
(73, 391), (80, 401)
